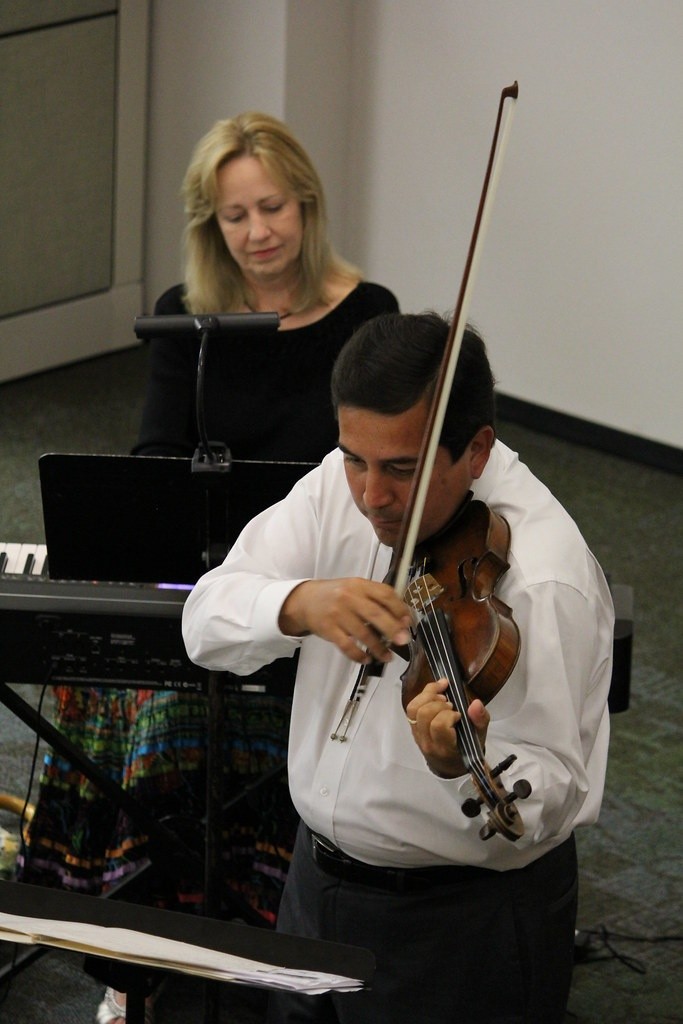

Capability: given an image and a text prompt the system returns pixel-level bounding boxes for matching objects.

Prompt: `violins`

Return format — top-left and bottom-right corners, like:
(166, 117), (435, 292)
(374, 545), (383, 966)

(383, 499), (534, 843)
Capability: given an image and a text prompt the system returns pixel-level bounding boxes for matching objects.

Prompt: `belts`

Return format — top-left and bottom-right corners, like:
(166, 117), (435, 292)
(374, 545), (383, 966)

(309, 831), (504, 890)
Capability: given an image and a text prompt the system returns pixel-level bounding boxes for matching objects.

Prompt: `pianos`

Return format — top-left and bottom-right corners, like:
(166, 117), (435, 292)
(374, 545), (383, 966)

(0, 574), (635, 1023)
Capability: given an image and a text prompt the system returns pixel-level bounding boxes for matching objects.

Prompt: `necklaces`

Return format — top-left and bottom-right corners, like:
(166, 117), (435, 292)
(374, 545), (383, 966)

(244, 296), (295, 321)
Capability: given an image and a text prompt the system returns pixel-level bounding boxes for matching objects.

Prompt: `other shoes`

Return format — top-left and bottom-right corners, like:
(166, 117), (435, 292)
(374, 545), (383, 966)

(96, 988), (158, 1023)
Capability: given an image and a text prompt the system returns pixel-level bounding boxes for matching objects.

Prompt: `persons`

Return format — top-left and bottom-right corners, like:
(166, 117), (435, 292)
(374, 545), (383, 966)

(18, 110), (407, 1024)
(182, 310), (620, 1024)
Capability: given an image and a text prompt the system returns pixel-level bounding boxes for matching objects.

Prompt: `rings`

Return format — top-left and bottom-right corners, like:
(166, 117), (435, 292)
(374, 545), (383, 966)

(406, 716), (418, 726)
